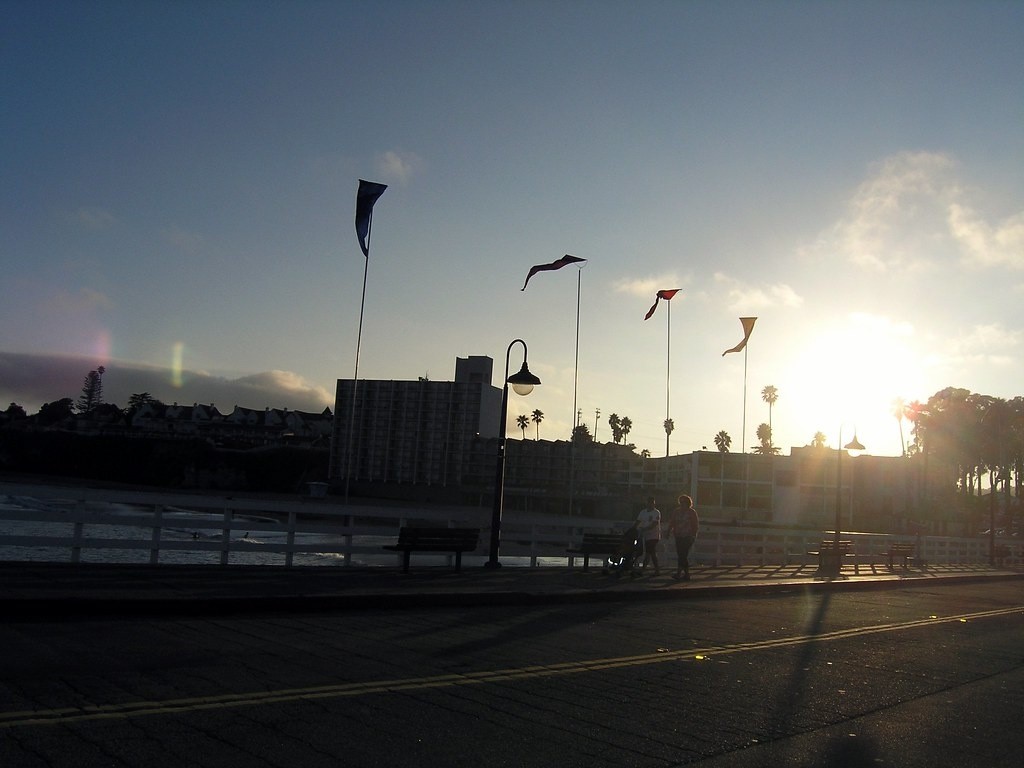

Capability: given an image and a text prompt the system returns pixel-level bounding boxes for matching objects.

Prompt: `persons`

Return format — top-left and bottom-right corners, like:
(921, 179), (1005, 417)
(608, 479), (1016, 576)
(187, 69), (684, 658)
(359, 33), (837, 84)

(666, 495), (699, 580)
(631, 496), (661, 577)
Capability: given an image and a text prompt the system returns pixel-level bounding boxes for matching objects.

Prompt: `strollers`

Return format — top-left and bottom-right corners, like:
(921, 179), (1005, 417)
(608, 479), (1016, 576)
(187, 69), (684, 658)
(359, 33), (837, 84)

(603, 524), (644, 579)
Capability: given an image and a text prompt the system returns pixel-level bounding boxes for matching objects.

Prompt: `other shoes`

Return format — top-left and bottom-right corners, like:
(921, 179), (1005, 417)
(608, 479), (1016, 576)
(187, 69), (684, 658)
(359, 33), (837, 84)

(679, 575), (690, 581)
(672, 573), (680, 578)
(650, 570), (660, 577)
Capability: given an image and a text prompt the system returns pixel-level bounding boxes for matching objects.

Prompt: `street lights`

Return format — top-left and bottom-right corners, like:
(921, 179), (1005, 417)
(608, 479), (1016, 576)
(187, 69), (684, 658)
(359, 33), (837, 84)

(989, 476), (1012, 566)
(831, 418), (866, 574)
(488, 339), (540, 566)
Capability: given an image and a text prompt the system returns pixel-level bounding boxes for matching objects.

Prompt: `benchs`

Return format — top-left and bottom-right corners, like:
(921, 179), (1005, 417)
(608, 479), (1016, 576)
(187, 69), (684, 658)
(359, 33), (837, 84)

(808, 541), (918, 572)
(566, 533), (643, 573)
(382, 526), (480, 574)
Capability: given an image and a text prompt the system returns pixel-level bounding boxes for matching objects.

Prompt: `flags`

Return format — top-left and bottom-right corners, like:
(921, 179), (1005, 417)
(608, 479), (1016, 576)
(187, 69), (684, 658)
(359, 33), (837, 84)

(520, 254), (588, 292)
(355, 179), (388, 258)
(644, 289), (679, 320)
(722, 317), (757, 357)
